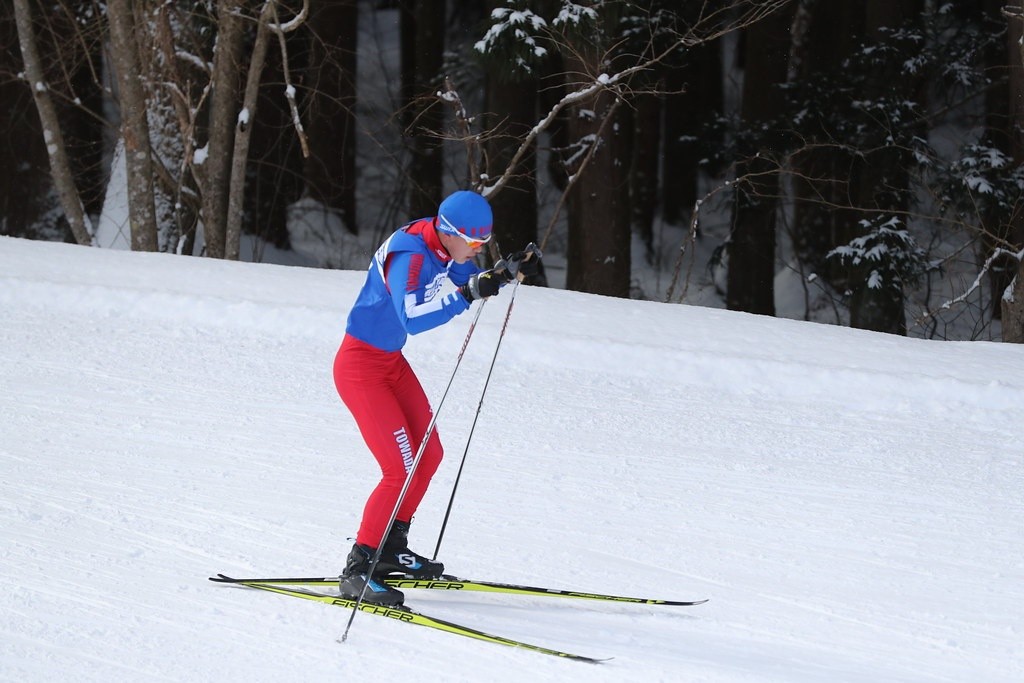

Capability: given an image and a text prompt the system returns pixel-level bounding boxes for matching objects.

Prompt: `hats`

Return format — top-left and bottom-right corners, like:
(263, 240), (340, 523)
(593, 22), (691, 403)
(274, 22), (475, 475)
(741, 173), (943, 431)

(435, 191), (493, 239)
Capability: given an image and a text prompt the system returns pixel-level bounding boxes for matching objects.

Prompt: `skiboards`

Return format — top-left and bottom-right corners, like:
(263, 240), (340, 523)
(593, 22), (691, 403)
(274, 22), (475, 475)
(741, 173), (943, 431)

(208, 574), (712, 665)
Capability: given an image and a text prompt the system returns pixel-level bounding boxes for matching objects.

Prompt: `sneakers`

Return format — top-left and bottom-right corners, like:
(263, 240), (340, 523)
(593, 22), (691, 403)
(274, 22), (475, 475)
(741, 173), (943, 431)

(338, 537), (404, 606)
(379, 516), (444, 580)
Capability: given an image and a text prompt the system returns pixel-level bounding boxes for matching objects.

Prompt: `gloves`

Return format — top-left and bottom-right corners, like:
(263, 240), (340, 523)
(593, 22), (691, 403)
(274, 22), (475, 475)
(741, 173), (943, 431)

(501, 242), (542, 283)
(461, 265), (505, 304)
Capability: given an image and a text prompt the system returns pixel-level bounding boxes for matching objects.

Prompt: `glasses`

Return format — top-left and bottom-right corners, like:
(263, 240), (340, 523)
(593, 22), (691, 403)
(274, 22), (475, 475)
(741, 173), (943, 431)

(441, 214), (491, 249)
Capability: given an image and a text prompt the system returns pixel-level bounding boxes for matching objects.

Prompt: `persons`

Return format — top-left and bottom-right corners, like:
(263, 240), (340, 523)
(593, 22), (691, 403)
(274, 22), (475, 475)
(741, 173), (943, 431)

(333, 190), (542, 606)
